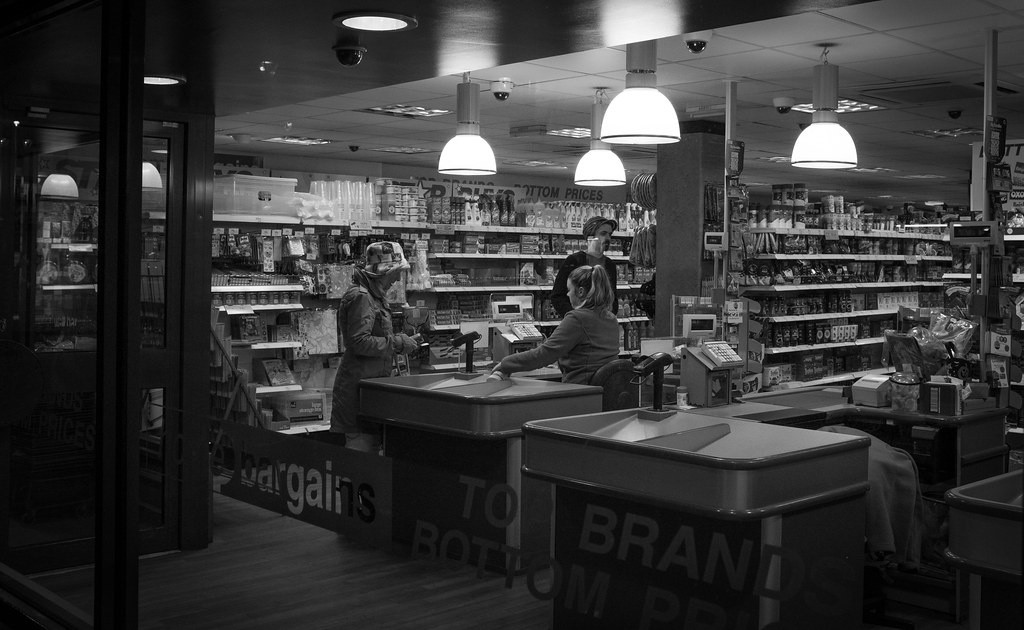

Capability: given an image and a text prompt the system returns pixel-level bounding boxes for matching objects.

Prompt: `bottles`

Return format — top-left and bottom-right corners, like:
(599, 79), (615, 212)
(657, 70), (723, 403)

(535, 296), (563, 346)
(747, 183), (862, 231)
(517, 197), (566, 229)
(616, 293), (655, 351)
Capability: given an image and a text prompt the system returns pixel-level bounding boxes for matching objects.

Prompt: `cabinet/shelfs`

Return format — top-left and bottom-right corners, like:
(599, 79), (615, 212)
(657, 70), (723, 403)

(41, 194), (1024, 427)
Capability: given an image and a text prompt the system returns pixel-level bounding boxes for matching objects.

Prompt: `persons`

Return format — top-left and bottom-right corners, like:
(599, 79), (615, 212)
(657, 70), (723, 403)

(328, 242), (419, 454)
(553, 215), (618, 316)
(491, 265), (620, 385)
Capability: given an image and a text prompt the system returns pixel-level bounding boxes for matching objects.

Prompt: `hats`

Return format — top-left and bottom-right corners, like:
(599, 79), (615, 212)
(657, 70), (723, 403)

(582, 216), (618, 239)
(363, 241), (411, 278)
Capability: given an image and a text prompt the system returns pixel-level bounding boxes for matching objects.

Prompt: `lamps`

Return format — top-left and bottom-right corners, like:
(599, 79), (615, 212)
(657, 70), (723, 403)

(789, 43), (859, 171)
(600, 37), (682, 144)
(142, 160), (163, 192)
(573, 87), (627, 186)
(39, 152), (79, 201)
(438, 72), (498, 176)
